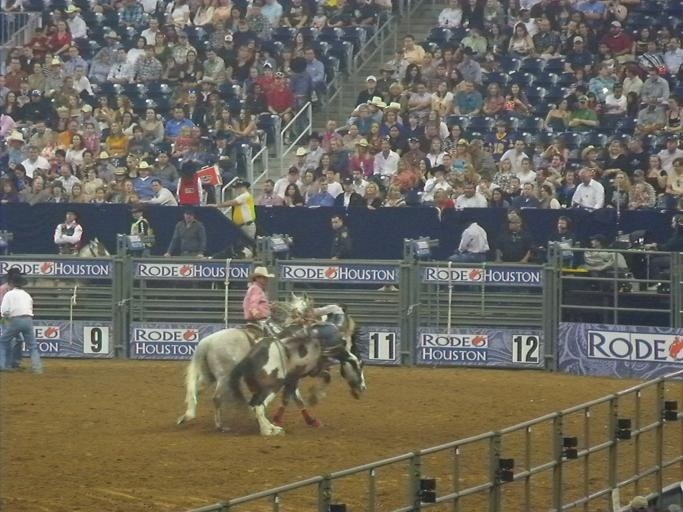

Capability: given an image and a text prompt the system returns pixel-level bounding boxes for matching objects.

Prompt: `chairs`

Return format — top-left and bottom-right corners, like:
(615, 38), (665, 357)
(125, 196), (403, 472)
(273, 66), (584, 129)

(331, 0), (682, 208)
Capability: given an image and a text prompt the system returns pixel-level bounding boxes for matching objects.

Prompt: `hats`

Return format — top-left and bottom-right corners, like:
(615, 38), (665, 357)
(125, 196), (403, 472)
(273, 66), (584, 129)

(6, 5), (285, 170)
(3, 267), (27, 286)
(293, 21), (677, 184)
(249, 266), (275, 280)
(232, 179), (251, 186)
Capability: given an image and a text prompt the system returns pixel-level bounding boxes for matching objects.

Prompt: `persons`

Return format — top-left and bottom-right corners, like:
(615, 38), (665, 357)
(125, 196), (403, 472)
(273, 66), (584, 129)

(0, 0), (683, 268)
(295, 303), (347, 346)
(243, 266), (278, 324)
(0, 268), (42, 375)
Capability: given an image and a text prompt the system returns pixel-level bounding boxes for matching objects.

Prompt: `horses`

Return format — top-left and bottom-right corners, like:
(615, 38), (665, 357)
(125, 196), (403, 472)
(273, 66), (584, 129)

(176, 290), (368, 438)
